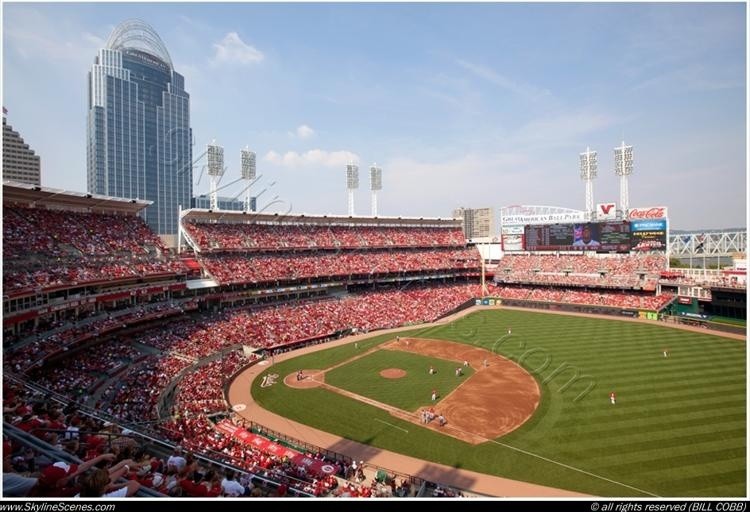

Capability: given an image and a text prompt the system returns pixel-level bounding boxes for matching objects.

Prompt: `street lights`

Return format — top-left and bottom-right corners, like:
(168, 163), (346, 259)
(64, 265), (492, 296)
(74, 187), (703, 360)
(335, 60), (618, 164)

(614, 141), (634, 220)
(344, 161), (359, 216)
(579, 146), (598, 219)
(206, 139), (226, 211)
(240, 146), (257, 211)
(368, 162), (382, 216)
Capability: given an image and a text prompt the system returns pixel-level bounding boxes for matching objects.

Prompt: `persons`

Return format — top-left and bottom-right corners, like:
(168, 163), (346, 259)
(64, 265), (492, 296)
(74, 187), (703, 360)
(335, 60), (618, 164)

(493, 253), (745, 292)
(574, 225), (601, 246)
(4, 206), (487, 497)
(609, 391), (616, 404)
(507, 326), (512, 337)
(664, 348), (669, 358)
(488, 285), (673, 311)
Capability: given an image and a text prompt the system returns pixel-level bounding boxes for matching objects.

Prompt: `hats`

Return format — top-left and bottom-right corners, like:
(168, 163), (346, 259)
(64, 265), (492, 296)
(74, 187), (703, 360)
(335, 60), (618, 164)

(36, 461), (78, 486)
(103, 421), (113, 427)
